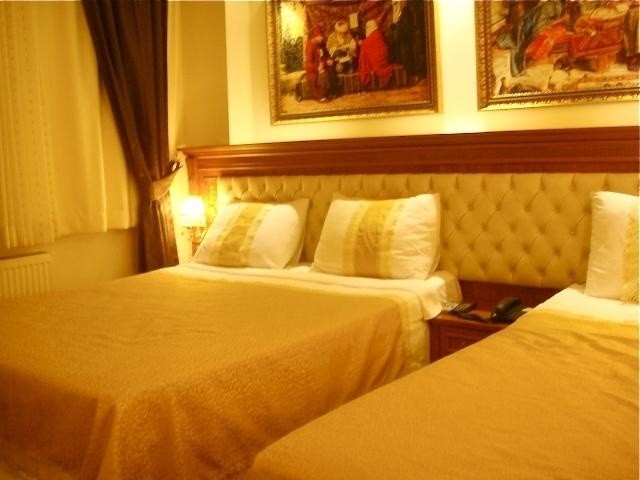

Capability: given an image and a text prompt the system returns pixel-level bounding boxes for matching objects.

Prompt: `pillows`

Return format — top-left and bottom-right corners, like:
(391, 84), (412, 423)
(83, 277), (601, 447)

(586, 189), (640, 300)
(190, 192), (445, 286)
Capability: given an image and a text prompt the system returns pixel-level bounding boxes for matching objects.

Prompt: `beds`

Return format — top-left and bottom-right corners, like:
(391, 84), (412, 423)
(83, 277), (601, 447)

(260, 282), (638, 480)
(0, 264), (462, 480)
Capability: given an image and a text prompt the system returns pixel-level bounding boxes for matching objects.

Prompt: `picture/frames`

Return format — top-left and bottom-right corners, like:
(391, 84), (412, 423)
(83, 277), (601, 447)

(474, 2), (637, 113)
(263, 0), (443, 125)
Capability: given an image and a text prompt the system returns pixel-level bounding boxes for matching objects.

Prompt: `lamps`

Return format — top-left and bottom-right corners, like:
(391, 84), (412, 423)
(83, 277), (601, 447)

(180, 194), (214, 246)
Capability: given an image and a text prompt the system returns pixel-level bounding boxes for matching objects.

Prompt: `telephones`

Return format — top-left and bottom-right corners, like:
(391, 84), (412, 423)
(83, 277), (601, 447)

(493, 296), (528, 323)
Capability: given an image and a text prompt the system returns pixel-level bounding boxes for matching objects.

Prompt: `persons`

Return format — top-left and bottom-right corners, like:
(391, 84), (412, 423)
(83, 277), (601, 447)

(307, 24), (338, 104)
(492, 0), (640, 76)
(352, 19), (390, 86)
(324, 21), (360, 73)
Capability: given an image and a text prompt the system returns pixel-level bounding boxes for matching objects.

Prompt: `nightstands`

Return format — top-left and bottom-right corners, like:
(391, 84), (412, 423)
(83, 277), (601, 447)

(429, 299), (515, 365)
